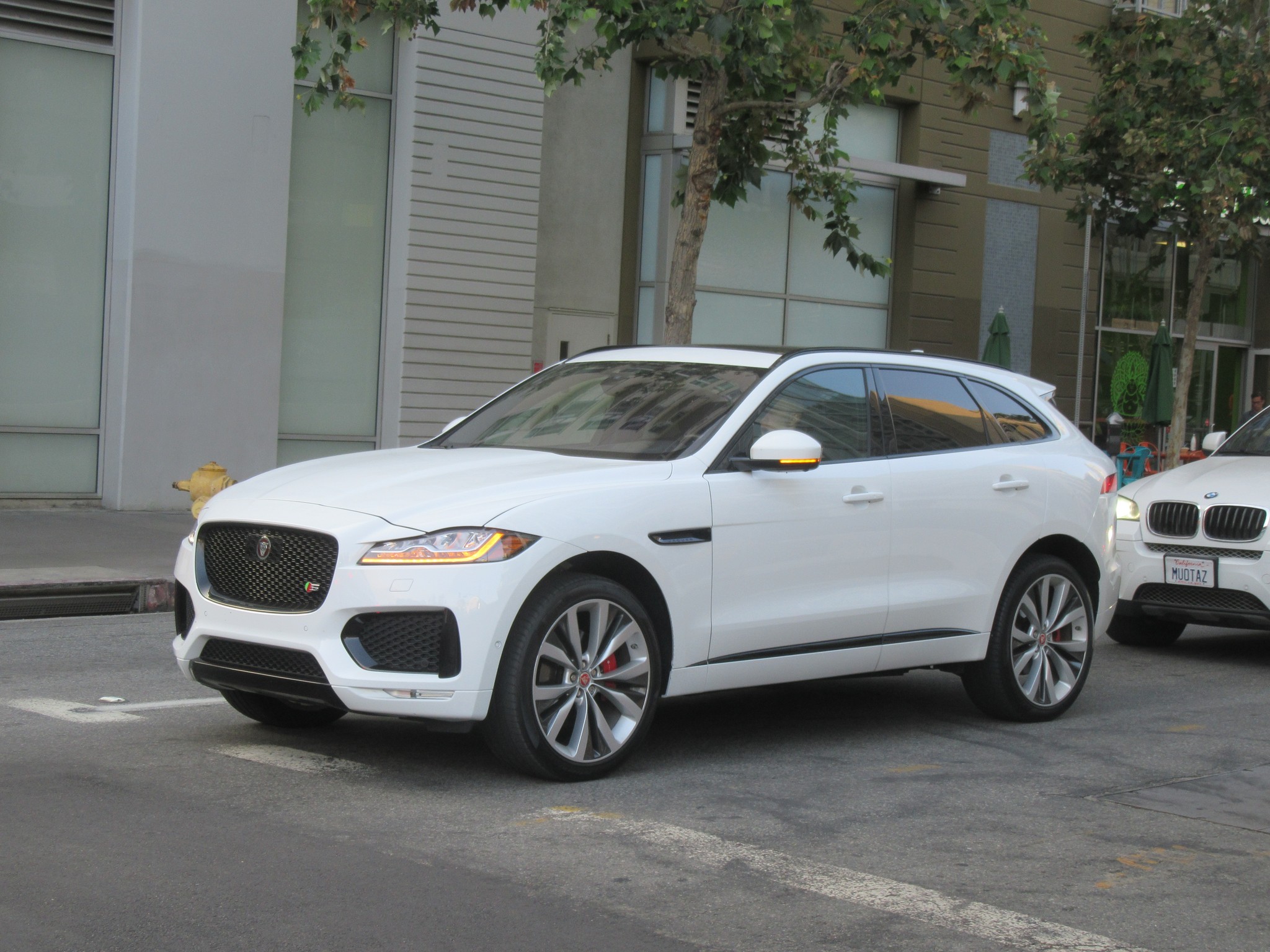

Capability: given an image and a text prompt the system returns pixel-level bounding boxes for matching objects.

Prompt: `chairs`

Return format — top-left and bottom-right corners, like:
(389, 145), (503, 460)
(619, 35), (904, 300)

(1116, 442), (1163, 490)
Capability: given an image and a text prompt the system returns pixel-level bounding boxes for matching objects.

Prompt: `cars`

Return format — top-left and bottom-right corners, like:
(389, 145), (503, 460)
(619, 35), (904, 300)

(1105, 402), (1270, 648)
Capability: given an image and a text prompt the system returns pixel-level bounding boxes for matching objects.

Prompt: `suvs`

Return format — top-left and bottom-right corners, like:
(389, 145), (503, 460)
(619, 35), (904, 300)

(174, 345), (1123, 785)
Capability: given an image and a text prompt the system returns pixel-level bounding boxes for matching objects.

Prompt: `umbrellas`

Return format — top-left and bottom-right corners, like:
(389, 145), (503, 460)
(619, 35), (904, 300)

(982, 304), (1012, 370)
(1143, 318), (1174, 473)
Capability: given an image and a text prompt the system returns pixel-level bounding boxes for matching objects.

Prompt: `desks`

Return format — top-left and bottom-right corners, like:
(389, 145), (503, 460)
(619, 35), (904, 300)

(1151, 450), (1205, 465)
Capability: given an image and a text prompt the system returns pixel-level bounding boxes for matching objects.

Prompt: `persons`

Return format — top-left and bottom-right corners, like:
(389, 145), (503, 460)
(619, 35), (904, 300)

(1237, 391), (1267, 429)
(758, 391), (804, 441)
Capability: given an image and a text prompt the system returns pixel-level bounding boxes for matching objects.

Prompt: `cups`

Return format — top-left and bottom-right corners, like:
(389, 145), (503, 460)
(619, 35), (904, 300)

(1180, 448), (1189, 453)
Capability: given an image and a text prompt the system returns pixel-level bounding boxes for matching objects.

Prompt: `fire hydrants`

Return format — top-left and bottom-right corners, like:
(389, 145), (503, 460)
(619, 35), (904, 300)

(171, 462), (238, 519)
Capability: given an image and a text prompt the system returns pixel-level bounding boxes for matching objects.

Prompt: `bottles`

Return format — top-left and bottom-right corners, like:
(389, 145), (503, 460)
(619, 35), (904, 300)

(1189, 434), (1196, 451)
